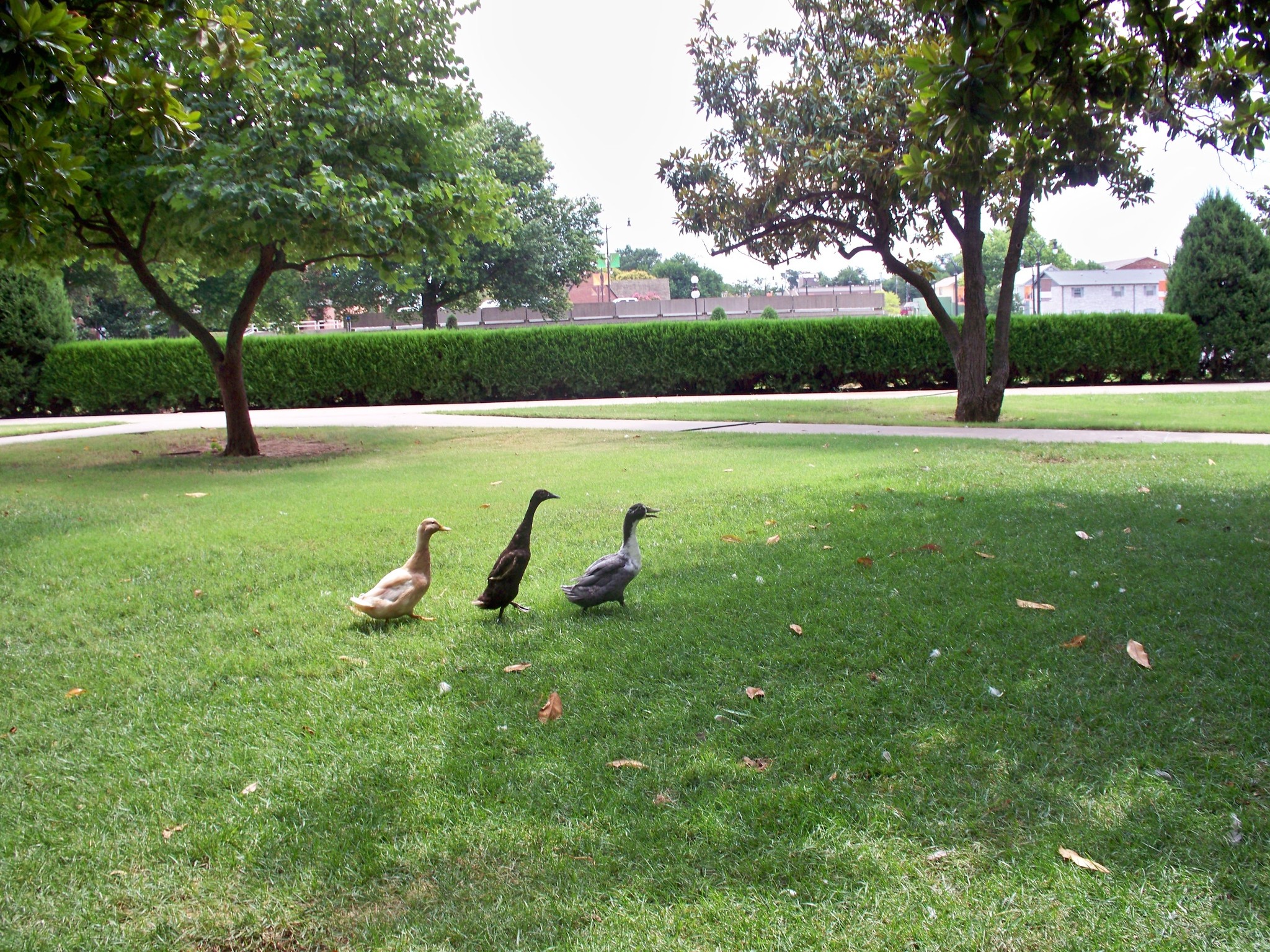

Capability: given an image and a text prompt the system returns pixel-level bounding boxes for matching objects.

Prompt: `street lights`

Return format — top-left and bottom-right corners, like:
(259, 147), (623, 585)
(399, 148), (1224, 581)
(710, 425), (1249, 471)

(1021, 238), (1058, 315)
(1020, 259), (1036, 314)
(939, 257), (963, 317)
(691, 275), (700, 320)
(848, 267), (880, 294)
(598, 216), (631, 302)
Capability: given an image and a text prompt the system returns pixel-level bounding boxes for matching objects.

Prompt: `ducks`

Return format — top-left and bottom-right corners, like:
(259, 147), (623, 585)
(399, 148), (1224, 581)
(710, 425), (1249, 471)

(561, 504), (661, 612)
(472, 489), (560, 624)
(350, 518), (451, 625)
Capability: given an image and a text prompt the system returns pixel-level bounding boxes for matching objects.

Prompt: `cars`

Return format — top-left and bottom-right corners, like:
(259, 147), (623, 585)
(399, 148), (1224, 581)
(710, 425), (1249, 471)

(612, 298), (638, 304)
